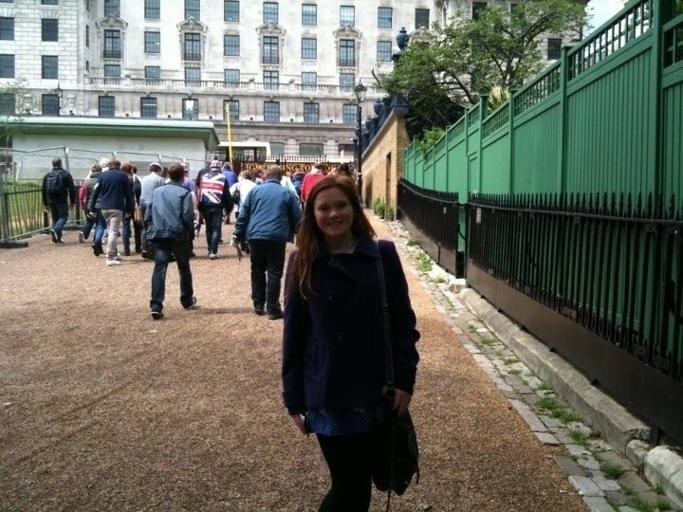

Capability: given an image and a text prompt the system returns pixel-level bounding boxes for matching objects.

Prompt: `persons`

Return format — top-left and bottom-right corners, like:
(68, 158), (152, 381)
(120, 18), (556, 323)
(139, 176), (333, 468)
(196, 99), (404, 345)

(78, 158), (350, 266)
(144, 164), (198, 319)
(281, 174), (420, 512)
(232, 164), (303, 320)
(42, 158), (76, 243)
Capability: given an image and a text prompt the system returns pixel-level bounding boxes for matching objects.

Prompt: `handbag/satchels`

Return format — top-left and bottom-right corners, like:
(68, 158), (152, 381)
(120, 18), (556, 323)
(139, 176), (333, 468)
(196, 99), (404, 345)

(79, 179), (88, 204)
(233, 185), (240, 205)
(365, 388), (420, 498)
(133, 205), (145, 227)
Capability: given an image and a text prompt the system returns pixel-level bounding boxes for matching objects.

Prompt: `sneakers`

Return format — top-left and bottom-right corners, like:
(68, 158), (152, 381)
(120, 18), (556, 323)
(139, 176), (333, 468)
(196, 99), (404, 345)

(268, 311), (284, 320)
(50, 228), (59, 244)
(208, 253), (218, 259)
(92, 240), (104, 256)
(151, 311), (164, 319)
(185, 296), (197, 309)
(255, 300), (264, 314)
(106, 249), (154, 266)
(79, 232), (85, 243)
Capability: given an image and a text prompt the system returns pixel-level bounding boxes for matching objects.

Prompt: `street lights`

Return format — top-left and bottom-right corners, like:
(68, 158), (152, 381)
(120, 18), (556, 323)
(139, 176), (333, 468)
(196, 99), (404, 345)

(354, 78), (367, 201)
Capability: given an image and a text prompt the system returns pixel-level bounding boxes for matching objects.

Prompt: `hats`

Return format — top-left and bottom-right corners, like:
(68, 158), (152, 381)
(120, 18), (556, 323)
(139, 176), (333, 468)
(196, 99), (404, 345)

(209, 160), (222, 168)
